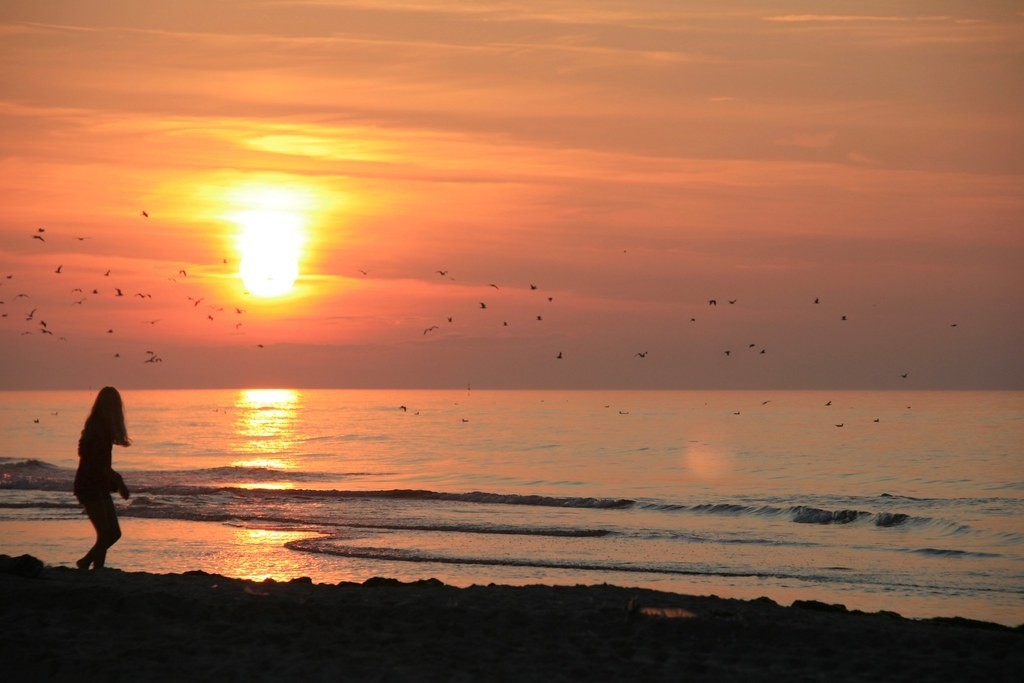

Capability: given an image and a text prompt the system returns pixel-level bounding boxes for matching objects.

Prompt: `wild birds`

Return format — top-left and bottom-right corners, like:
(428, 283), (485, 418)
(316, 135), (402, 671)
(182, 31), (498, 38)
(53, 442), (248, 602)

(874, 418), (879, 422)
(902, 374), (907, 377)
(462, 419), (468, 422)
(835, 423), (843, 427)
(557, 352), (562, 358)
(360, 270), (369, 274)
(724, 344), (766, 356)
(842, 316), (847, 320)
(0, 228), (263, 425)
(762, 401), (770, 405)
(690, 300), (736, 321)
(399, 269), (553, 335)
(141, 211), (148, 217)
(951, 324), (957, 326)
(825, 400), (832, 406)
(400, 405), (419, 415)
(635, 351), (648, 357)
(813, 297), (821, 304)
(733, 411), (740, 414)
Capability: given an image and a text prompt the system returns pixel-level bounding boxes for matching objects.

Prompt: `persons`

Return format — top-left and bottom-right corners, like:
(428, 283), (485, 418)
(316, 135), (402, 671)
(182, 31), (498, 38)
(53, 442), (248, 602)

(74, 386), (131, 573)
